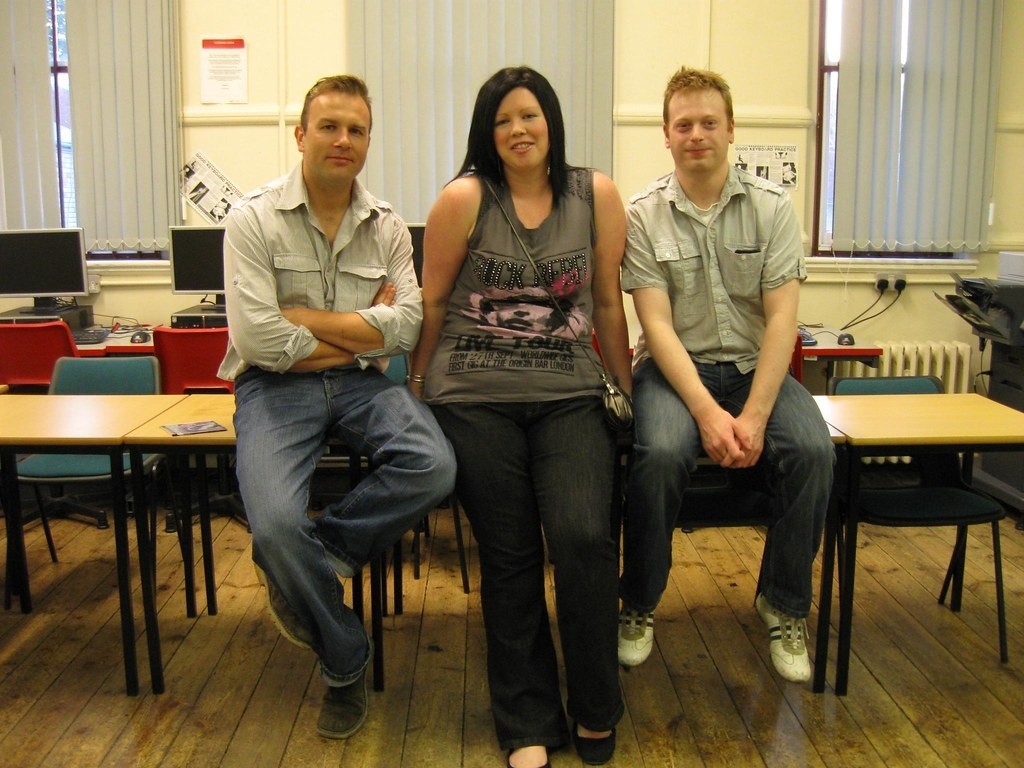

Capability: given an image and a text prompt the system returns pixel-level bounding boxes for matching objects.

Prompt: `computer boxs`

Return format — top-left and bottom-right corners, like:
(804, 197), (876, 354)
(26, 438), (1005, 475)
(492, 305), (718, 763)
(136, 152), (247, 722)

(170, 305), (228, 329)
(0, 305), (94, 331)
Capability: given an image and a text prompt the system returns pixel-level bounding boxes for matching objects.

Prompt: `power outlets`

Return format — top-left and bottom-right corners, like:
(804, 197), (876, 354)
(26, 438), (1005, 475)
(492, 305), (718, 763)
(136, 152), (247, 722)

(875, 271), (906, 292)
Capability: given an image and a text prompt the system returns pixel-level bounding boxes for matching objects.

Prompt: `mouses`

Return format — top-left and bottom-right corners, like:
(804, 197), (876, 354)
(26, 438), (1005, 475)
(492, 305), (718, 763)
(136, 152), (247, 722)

(838, 334), (854, 345)
(130, 331), (151, 343)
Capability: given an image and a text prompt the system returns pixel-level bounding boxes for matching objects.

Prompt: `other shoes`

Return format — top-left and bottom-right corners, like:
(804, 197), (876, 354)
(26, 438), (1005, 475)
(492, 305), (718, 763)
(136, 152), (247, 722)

(506, 748), (550, 768)
(572, 721), (616, 764)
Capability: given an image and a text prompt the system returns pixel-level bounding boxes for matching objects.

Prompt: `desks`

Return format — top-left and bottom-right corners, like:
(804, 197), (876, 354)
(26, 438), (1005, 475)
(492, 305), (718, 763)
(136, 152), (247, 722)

(590, 325), (883, 378)
(75, 320), (155, 358)
(0, 393), (1024, 698)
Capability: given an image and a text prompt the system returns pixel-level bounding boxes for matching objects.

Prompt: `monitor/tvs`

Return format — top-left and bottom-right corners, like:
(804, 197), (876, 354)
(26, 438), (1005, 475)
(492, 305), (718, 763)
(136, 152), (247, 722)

(406, 222), (427, 290)
(169, 226), (228, 309)
(0, 227), (89, 311)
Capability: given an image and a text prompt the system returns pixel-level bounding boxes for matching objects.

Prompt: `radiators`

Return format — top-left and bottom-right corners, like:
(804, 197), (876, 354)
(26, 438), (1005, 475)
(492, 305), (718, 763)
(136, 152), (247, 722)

(834, 338), (973, 464)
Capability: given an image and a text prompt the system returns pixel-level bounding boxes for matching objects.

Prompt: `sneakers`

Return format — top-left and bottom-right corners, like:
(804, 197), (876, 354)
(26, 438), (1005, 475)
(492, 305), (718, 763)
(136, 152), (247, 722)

(254, 564), (313, 648)
(617, 601), (656, 665)
(754, 593), (811, 683)
(317, 669), (368, 739)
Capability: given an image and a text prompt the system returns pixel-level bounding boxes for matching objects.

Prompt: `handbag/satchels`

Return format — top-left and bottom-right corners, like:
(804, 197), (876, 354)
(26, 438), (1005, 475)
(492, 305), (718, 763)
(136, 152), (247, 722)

(602, 385), (635, 433)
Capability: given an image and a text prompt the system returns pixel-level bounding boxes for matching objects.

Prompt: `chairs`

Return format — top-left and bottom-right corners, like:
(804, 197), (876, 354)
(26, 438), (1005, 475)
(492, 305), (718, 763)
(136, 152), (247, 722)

(0, 330), (1008, 697)
(0, 320), (112, 529)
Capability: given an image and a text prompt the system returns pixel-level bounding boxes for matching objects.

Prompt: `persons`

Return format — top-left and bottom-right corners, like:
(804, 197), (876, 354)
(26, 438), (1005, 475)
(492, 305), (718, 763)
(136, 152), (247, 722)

(618, 66), (836, 683)
(216, 74), (458, 740)
(406, 66), (633, 768)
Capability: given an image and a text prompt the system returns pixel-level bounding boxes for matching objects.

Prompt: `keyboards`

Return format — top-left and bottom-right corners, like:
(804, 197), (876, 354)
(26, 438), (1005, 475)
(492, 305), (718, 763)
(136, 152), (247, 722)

(798, 329), (817, 346)
(70, 329), (111, 344)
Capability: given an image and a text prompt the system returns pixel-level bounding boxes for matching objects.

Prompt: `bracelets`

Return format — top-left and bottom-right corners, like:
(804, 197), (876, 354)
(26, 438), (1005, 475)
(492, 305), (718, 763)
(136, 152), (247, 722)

(409, 373), (427, 384)
